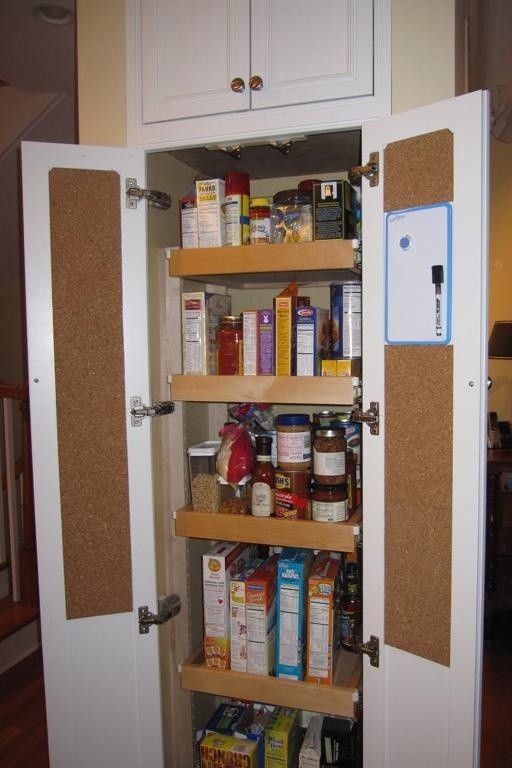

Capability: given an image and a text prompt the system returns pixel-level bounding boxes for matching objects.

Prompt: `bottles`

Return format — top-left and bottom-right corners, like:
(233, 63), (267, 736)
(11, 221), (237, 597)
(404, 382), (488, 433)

(249, 434), (275, 517)
(339, 559), (363, 654)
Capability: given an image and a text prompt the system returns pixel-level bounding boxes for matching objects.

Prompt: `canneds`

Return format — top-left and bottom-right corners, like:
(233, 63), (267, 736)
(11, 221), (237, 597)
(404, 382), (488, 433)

(274, 410), (362, 523)
(250, 198), (273, 245)
(340, 593), (362, 654)
